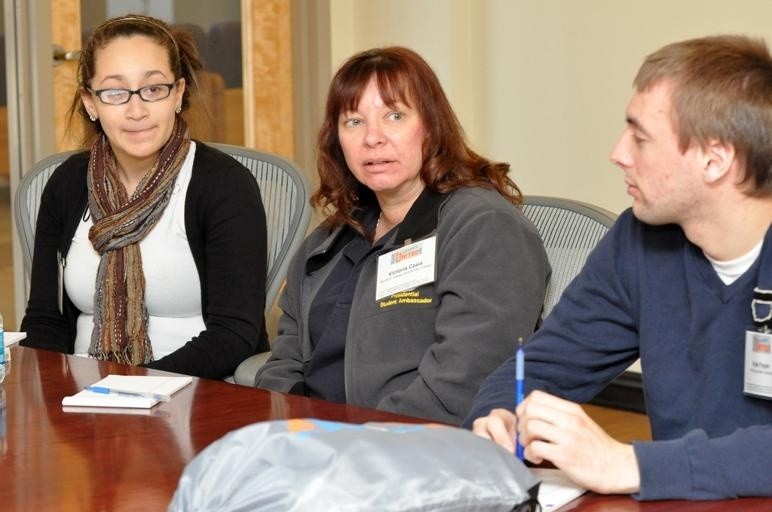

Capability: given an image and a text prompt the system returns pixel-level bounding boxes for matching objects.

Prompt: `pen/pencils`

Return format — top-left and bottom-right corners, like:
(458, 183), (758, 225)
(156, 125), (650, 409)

(514, 336), (525, 461)
(84, 385), (172, 402)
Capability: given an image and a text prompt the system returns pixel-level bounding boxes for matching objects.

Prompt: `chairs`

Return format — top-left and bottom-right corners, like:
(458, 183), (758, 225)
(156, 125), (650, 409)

(234, 189), (619, 389)
(13, 141), (309, 332)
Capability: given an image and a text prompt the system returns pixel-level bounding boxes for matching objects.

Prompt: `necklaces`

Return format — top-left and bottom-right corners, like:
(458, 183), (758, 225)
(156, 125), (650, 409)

(375, 212), (383, 230)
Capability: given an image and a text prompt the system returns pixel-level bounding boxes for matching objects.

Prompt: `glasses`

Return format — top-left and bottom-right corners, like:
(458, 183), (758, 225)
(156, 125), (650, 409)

(86, 80), (180, 107)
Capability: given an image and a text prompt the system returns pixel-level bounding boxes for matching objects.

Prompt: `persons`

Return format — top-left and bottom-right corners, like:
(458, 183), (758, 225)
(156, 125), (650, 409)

(253, 46), (553, 431)
(17, 13), (272, 391)
(457, 33), (770, 502)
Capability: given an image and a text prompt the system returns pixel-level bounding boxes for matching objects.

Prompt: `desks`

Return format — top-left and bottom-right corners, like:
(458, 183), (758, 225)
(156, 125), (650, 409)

(0, 331), (772, 512)
